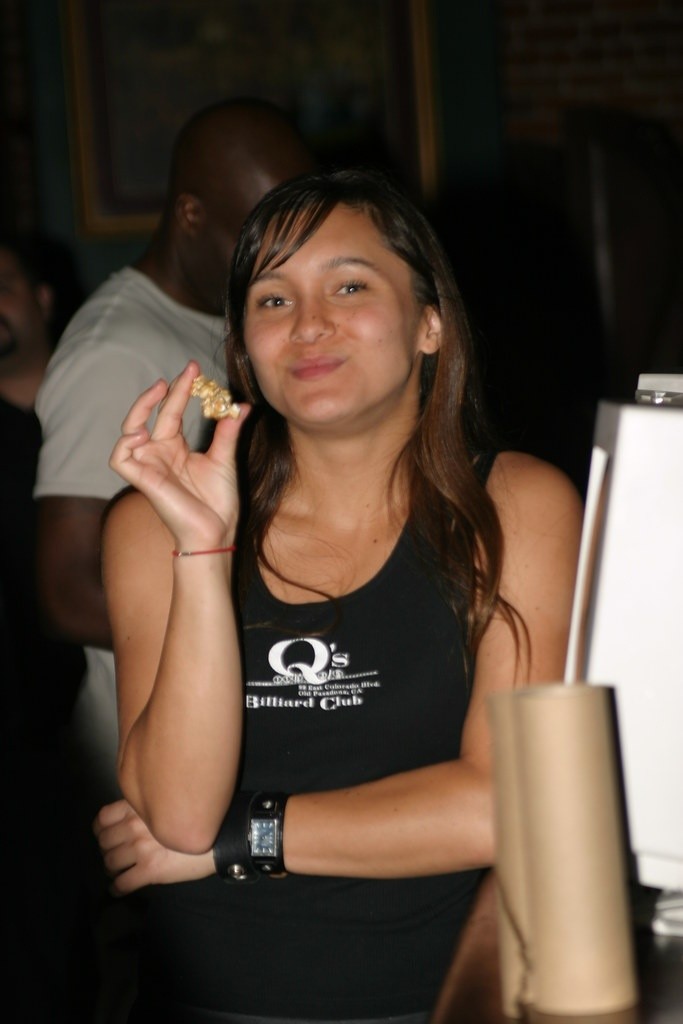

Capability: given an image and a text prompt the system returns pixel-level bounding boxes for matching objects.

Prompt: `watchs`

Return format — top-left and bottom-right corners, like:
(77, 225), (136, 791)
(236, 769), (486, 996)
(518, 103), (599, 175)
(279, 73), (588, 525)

(247, 794), (290, 879)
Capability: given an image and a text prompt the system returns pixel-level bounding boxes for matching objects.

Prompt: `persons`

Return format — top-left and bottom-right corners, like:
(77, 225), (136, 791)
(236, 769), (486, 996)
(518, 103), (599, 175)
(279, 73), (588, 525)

(93, 168), (583, 1024)
(27, 99), (312, 804)
(0, 248), (51, 842)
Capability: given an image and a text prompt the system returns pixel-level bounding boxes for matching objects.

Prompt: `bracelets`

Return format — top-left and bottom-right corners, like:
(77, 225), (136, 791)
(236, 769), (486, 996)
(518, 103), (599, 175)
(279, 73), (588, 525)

(172, 546), (236, 556)
(214, 791), (263, 886)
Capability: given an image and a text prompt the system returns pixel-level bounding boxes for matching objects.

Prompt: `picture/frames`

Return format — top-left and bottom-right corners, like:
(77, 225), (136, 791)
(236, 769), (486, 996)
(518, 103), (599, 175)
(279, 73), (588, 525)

(57, 1), (448, 239)
